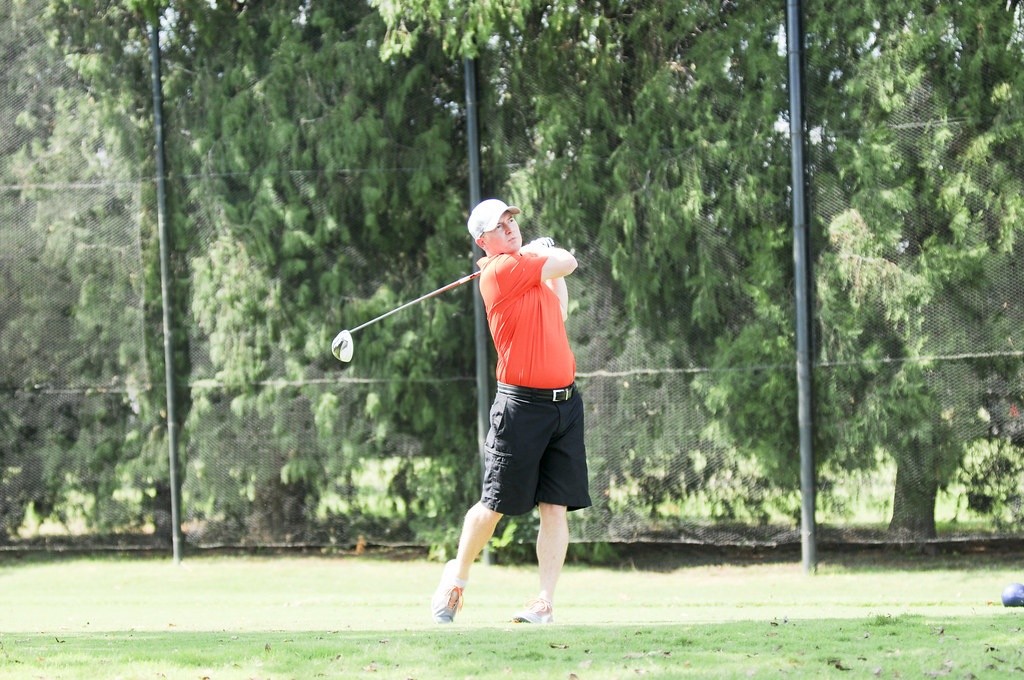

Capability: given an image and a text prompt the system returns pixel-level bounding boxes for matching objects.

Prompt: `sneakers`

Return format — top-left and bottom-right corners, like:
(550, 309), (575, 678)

(512, 598), (554, 624)
(432, 559), (468, 625)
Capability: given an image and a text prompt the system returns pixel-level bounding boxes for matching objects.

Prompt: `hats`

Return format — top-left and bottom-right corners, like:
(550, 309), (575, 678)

(467, 199), (520, 240)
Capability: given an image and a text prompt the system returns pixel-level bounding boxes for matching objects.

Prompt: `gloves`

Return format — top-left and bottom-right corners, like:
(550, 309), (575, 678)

(530, 236), (555, 248)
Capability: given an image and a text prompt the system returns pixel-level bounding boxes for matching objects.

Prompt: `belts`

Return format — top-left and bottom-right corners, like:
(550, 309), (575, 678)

(497, 381), (576, 402)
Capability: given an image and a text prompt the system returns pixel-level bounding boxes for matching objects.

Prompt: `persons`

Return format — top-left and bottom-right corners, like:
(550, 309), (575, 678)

(433, 199), (591, 625)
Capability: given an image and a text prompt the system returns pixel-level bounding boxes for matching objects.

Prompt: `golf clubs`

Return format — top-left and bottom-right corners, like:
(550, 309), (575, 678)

(330, 270), (482, 364)
